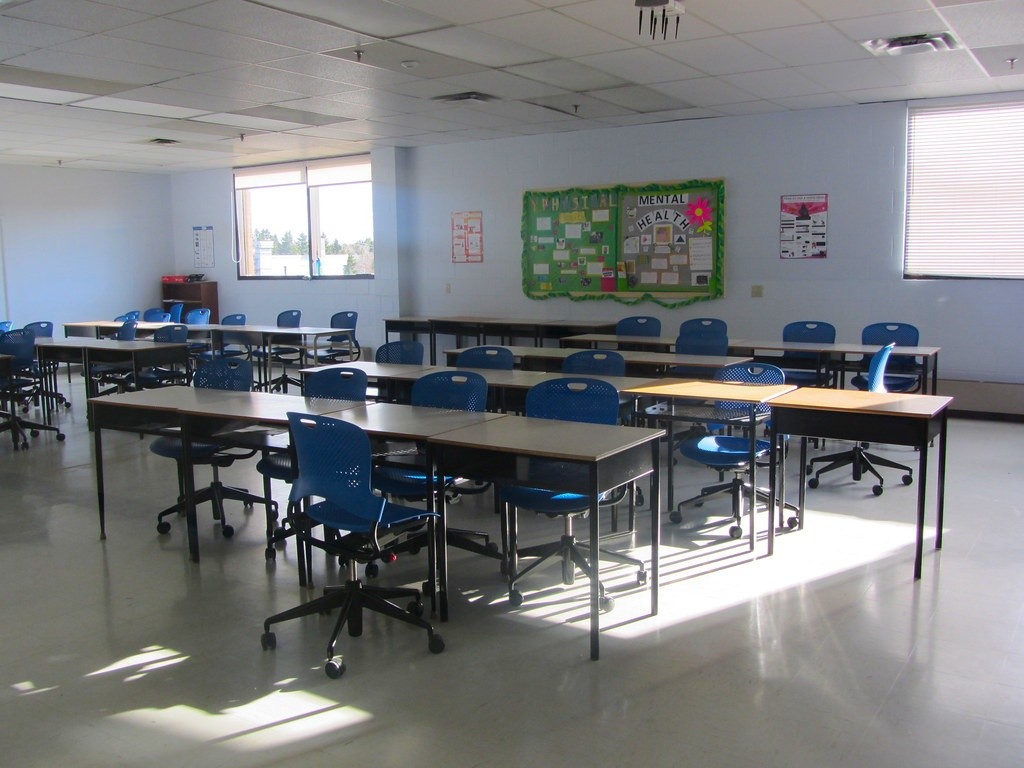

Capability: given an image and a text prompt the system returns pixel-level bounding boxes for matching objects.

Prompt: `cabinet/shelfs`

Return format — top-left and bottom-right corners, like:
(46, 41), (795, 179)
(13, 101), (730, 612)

(163, 281), (219, 325)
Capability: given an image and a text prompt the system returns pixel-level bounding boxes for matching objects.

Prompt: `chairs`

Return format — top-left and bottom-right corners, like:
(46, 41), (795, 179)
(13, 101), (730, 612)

(259, 411), (442, 677)
(0, 319), (72, 450)
(498, 378), (648, 614)
(34, 302), (921, 562)
(365, 372), (514, 584)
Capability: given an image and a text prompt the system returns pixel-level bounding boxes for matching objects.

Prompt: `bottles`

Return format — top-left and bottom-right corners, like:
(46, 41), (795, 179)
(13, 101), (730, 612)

(316, 258), (321, 276)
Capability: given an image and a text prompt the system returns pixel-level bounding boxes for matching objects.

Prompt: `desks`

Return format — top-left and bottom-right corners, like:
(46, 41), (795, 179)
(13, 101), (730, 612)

(429, 316), (509, 366)
(382, 317), (458, 366)
(35, 335), (110, 427)
(211, 326), (288, 394)
(82, 330), (957, 661)
(265, 326), (354, 393)
(84, 339), (188, 432)
(537, 316), (615, 349)
(162, 322), (236, 389)
(482, 318), (564, 347)
(61, 321), (116, 384)
(96, 320), (175, 340)
